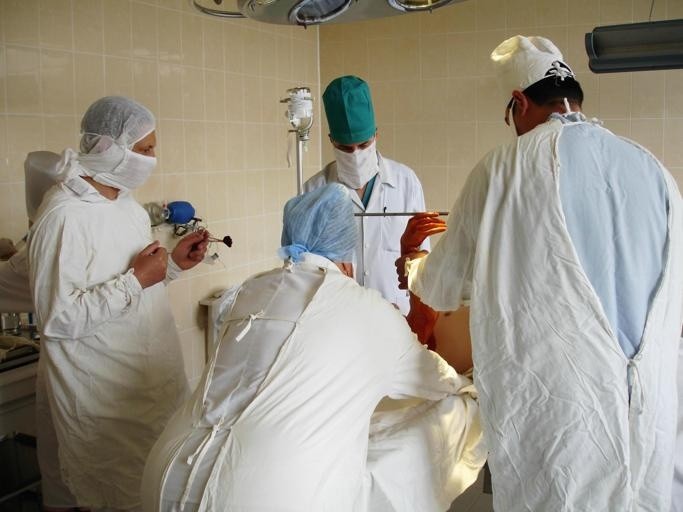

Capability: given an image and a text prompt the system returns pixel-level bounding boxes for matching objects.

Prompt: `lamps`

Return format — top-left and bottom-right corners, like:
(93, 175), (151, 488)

(584, 0), (683, 72)
(193, 0), (465, 29)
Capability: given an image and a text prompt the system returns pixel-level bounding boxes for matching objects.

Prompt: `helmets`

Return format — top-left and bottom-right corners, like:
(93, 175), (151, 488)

(322, 74), (378, 147)
(490, 35), (577, 123)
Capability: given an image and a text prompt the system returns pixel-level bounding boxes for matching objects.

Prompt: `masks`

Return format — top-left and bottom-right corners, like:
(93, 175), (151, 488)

(330, 140), (380, 191)
(506, 99), (520, 144)
(110, 139), (159, 195)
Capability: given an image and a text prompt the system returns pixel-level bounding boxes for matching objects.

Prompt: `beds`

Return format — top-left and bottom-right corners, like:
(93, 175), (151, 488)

(197, 250), (607, 508)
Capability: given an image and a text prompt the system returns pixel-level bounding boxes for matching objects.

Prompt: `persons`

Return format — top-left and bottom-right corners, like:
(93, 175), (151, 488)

(28, 97), (211, 511)
(0, 150), (71, 511)
(141, 183), (488, 510)
(406, 43), (680, 511)
(394, 211), (474, 374)
(298, 123), (430, 320)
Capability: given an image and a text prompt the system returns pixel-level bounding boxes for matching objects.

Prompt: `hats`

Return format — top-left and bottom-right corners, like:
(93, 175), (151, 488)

(278, 182), (359, 265)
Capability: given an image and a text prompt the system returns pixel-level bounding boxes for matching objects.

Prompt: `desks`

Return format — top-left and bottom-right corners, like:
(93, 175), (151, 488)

(0, 348), (42, 443)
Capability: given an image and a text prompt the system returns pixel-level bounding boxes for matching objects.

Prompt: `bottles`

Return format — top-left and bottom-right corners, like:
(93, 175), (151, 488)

(8, 312), (23, 336)
(283, 86), (315, 142)
